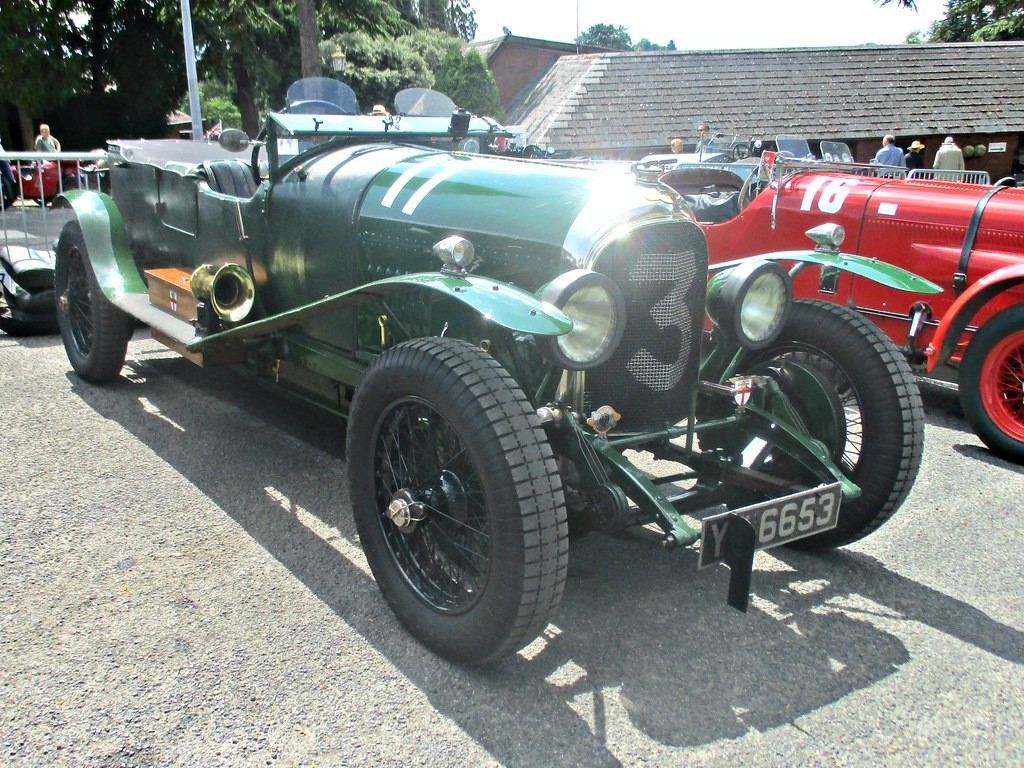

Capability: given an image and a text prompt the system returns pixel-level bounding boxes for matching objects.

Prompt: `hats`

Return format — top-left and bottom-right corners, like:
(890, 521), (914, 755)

(906, 140), (925, 153)
(941, 136), (955, 144)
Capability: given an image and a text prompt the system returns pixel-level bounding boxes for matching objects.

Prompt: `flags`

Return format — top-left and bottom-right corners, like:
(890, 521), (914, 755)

(204, 123), (221, 138)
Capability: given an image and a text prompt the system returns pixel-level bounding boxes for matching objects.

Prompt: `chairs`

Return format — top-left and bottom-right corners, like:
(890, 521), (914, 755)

(203, 155), (260, 198)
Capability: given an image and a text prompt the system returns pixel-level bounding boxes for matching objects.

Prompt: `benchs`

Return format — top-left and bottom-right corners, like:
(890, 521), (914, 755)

(684, 190), (749, 223)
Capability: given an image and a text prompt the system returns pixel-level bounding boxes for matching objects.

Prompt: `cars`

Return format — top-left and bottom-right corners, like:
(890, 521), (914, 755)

(619, 148), (1024, 464)
(5, 150), (110, 211)
(44, 75), (923, 670)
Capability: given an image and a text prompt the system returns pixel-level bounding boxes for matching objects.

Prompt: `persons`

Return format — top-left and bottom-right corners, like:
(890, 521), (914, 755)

(367, 105), (390, 116)
(671, 139), (685, 154)
(695, 124), (714, 153)
(932, 137), (965, 183)
(874, 135), (906, 179)
(34, 124), (60, 164)
(904, 141), (927, 179)
